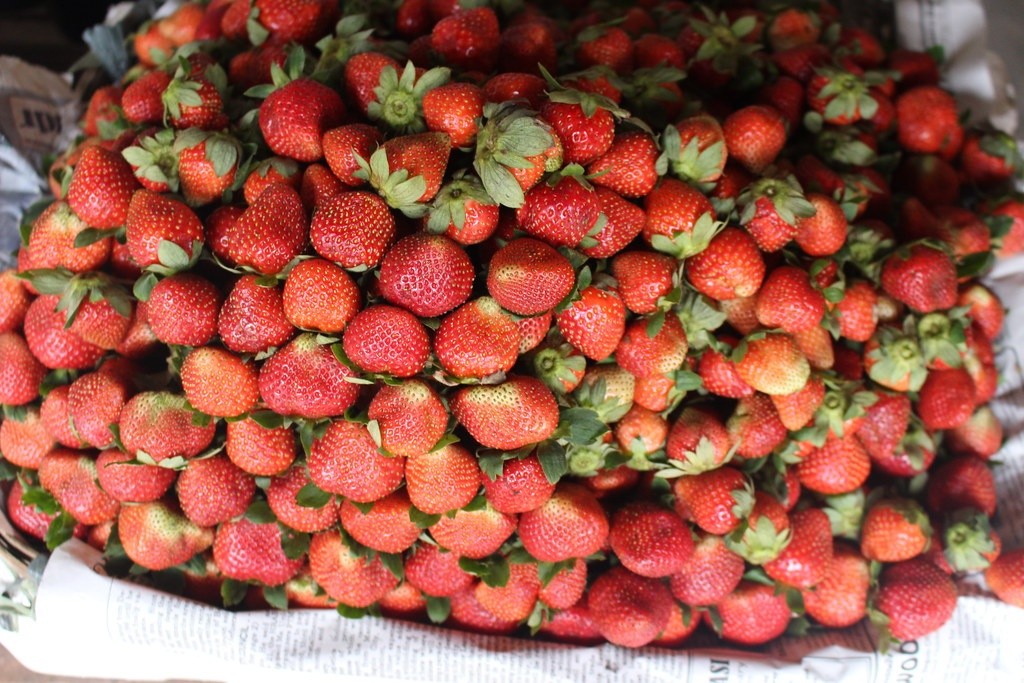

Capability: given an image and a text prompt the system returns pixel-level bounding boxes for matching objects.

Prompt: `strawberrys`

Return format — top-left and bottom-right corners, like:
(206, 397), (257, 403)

(0, 0), (1024, 649)
